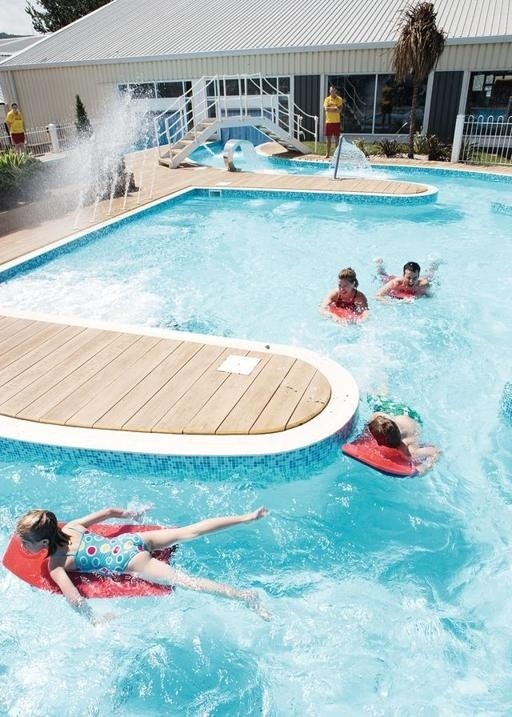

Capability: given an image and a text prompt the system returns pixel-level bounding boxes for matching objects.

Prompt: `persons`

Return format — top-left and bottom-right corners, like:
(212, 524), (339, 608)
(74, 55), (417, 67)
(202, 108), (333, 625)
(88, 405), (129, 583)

(380, 79), (395, 129)
(322, 83), (344, 159)
(17, 503), (272, 633)
(366, 411), (442, 477)
(371, 255), (448, 306)
(6, 102), (29, 159)
(320, 266), (371, 325)
